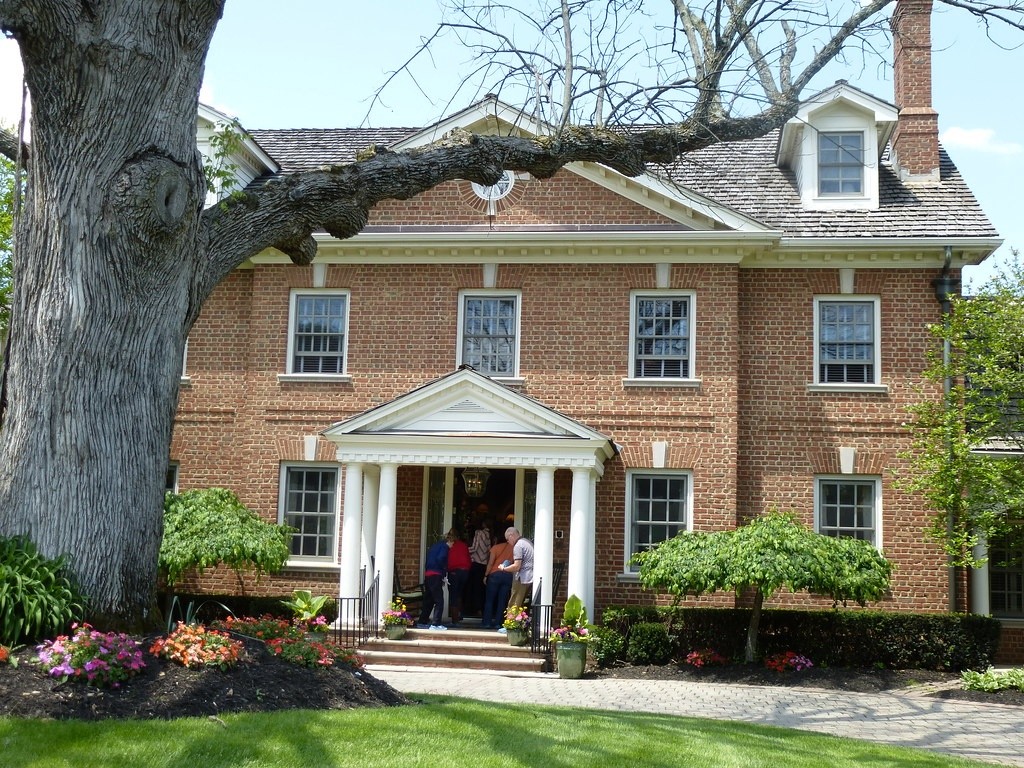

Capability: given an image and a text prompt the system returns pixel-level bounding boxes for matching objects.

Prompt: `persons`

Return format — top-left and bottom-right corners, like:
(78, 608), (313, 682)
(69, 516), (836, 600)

(415, 536), (456, 631)
(449, 518), (514, 629)
(499, 528), (534, 633)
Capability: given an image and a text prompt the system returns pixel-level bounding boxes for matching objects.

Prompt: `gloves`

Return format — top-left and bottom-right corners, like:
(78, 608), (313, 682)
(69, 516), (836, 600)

(498, 564), (504, 572)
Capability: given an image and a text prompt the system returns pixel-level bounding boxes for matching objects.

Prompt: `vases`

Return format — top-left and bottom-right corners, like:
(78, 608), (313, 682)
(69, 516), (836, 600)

(555, 643), (588, 680)
(507, 630), (527, 647)
(303, 632), (325, 644)
(384, 624), (407, 641)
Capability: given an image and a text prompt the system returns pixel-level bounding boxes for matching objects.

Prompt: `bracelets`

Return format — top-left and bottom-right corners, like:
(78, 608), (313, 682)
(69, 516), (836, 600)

(484, 574), (488, 578)
(502, 568), (505, 573)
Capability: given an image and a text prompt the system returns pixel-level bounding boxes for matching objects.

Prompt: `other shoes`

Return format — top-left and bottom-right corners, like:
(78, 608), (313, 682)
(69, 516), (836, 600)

(497, 627), (507, 634)
(447, 616), (464, 628)
(416, 623), (430, 629)
(479, 622), (490, 628)
(494, 624), (501, 629)
(429, 624), (448, 630)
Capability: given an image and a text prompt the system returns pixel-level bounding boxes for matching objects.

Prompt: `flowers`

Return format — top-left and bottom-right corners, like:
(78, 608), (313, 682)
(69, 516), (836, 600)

(686, 648), (813, 672)
(548, 593), (597, 643)
(500, 606), (531, 629)
(0, 616), (364, 688)
(381, 596), (414, 625)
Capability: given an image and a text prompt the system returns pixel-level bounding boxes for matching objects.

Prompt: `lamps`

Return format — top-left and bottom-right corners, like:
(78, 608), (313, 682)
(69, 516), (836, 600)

(461, 467), (490, 499)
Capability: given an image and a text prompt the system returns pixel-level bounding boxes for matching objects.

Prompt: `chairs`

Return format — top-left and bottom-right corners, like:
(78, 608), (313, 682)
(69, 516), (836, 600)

(521, 562), (564, 615)
(393, 560), (424, 619)
